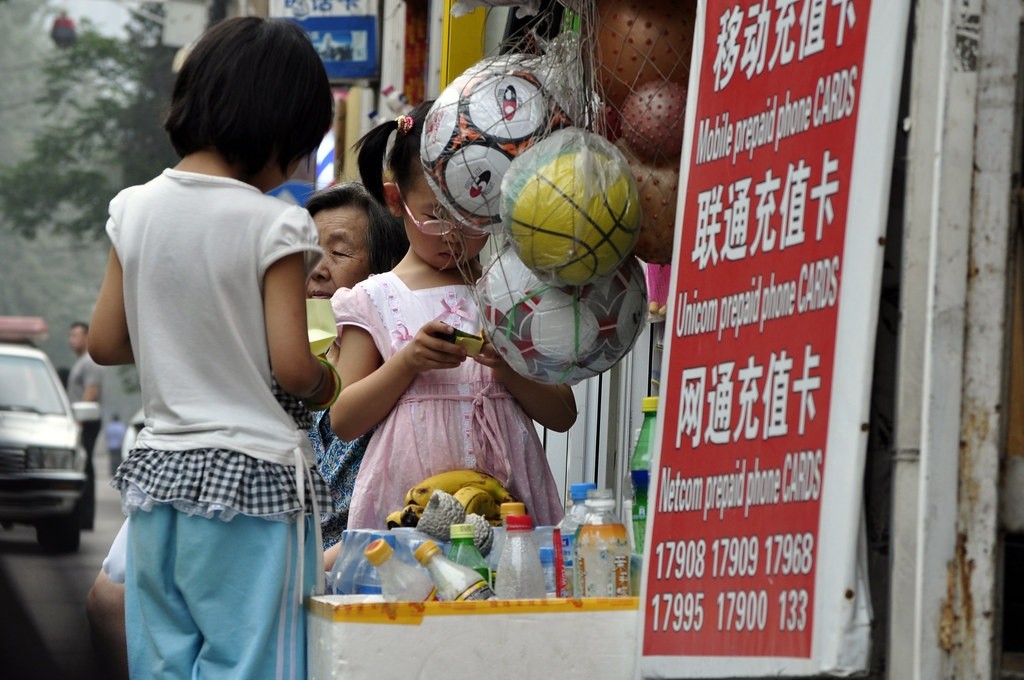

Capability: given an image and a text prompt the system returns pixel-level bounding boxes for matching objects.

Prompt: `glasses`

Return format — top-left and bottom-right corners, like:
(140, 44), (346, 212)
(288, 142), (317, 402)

(394, 180), (498, 239)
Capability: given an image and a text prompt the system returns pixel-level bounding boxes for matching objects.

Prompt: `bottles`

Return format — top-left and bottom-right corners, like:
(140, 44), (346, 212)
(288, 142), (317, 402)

(630, 396), (660, 555)
(326, 482), (632, 601)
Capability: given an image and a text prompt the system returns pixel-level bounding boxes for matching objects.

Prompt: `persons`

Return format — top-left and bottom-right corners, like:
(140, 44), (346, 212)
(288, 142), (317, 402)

(87, 187), (409, 648)
(330, 102), (577, 530)
(67, 321), (102, 531)
(105, 413), (125, 475)
(87, 17), (343, 680)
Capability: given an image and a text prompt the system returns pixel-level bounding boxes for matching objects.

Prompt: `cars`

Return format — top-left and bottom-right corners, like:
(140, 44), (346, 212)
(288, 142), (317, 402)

(1, 317), (103, 553)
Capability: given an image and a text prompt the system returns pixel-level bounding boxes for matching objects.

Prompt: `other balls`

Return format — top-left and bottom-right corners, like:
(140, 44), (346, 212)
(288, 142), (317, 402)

(472, 234), (649, 381)
(496, 135), (640, 287)
(422, 49), (581, 224)
